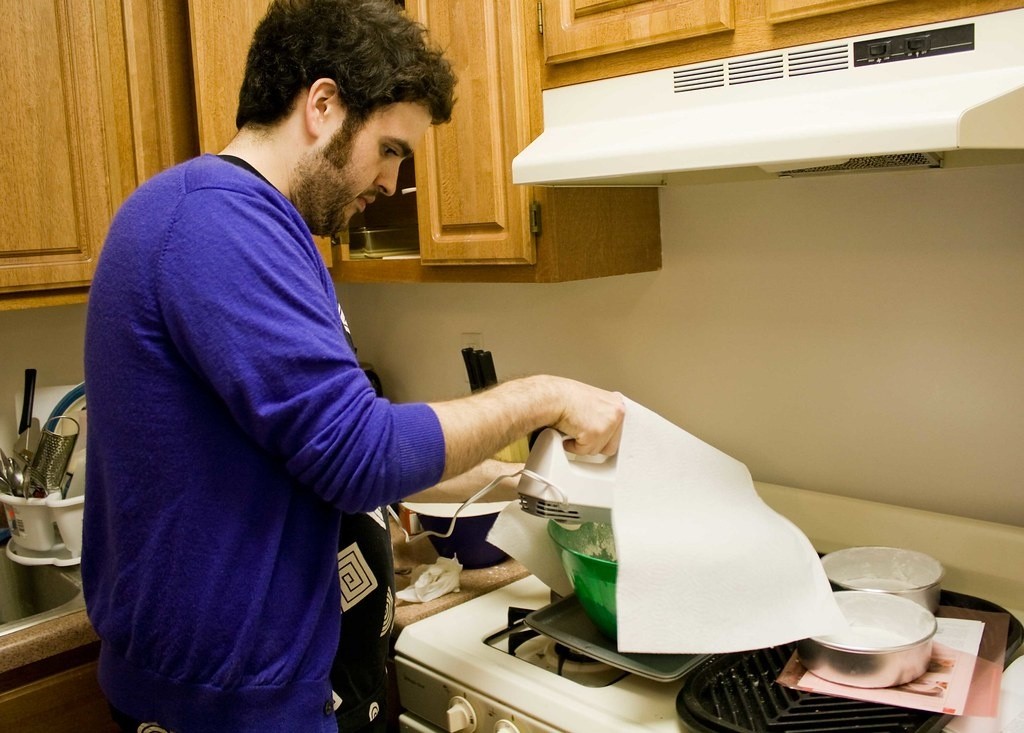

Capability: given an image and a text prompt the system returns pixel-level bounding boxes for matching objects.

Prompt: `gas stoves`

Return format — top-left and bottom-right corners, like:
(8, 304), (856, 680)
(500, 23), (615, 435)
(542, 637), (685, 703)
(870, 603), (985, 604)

(394, 574), (694, 733)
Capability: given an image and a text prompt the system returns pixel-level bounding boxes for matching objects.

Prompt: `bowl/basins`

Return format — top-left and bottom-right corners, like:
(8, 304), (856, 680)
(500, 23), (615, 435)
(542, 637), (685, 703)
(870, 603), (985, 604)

(401, 501), (513, 567)
(547, 519), (617, 639)
(820, 546), (945, 613)
(798, 591), (938, 690)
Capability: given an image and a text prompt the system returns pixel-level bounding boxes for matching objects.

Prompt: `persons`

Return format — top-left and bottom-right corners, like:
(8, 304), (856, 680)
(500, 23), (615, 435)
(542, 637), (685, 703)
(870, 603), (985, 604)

(78, 3), (625, 731)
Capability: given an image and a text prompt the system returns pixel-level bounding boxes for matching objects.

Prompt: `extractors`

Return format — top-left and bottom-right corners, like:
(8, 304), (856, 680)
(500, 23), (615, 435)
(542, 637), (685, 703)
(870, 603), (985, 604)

(510, 7), (1023, 188)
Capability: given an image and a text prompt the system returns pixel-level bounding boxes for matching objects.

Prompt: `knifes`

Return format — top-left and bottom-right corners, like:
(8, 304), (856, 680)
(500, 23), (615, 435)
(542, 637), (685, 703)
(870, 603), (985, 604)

(13, 368), (37, 460)
(0, 449), (32, 501)
